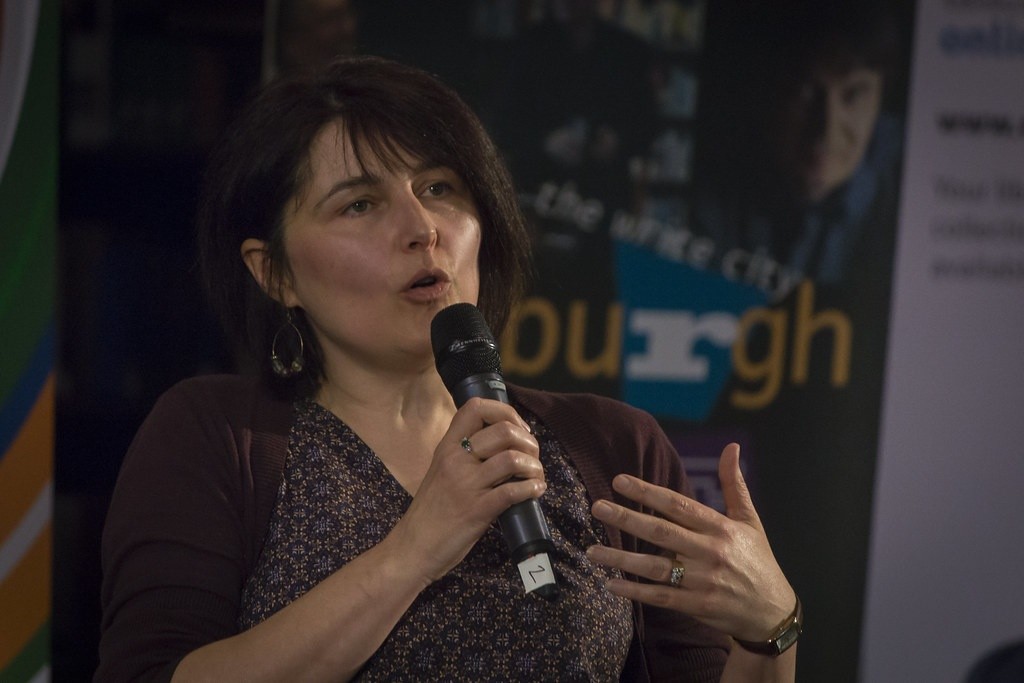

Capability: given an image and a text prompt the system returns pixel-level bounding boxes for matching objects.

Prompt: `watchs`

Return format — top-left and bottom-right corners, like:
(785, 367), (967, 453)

(732, 593), (804, 657)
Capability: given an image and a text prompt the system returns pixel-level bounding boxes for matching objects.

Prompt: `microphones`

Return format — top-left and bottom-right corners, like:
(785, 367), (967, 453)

(429, 301), (563, 603)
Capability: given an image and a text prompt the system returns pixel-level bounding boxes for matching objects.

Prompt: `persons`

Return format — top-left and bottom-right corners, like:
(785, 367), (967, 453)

(694, 0), (905, 284)
(94, 58), (804, 683)
(178, 0), (358, 147)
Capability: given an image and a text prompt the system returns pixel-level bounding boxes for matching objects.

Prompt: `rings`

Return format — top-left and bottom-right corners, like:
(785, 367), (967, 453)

(460, 436), (483, 463)
(667, 560), (684, 587)
